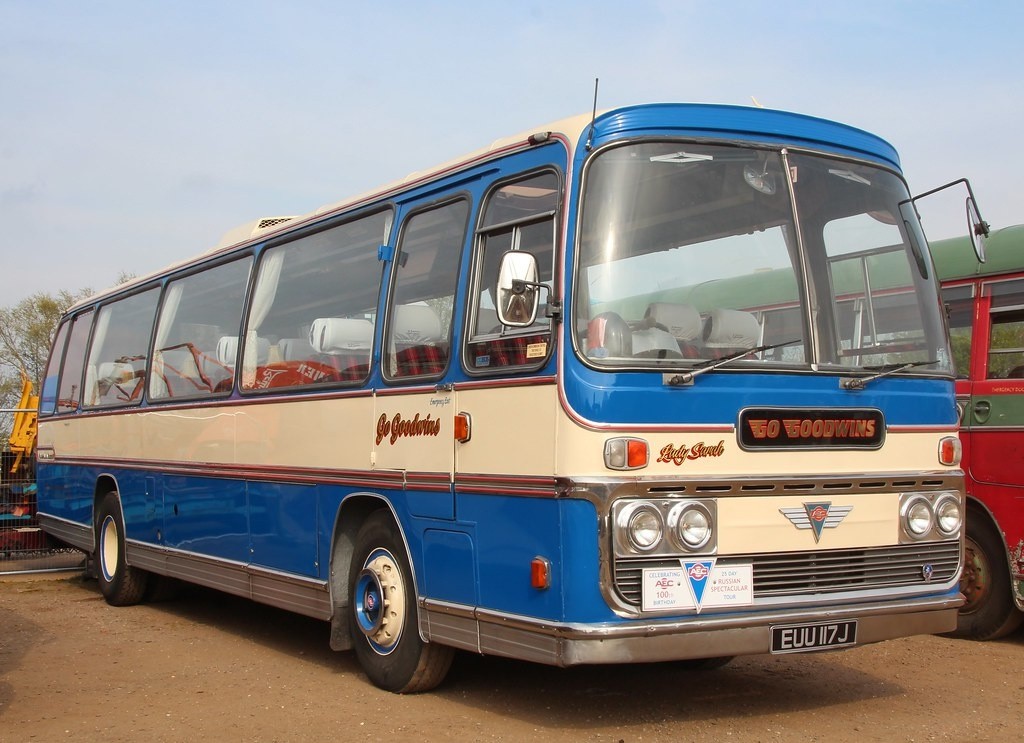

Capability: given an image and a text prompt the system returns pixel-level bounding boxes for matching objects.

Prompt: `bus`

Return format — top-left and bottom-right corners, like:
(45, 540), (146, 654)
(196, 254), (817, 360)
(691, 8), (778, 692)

(534, 223), (1024, 641)
(36, 104), (991, 694)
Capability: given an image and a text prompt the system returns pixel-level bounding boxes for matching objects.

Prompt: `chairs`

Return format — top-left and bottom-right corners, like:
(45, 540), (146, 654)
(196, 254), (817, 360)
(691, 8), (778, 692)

(214, 336), (269, 390)
(98, 362), (144, 404)
(1008, 365), (1024, 379)
(309, 318), (374, 382)
(641, 303), (702, 358)
(449, 309), (502, 368)
(700, 309), (760, 360)
(486, 285), (552, 366)
(277, 339), (319, 385)
(390, 305), (446, 377)
(198, 352), (233, 393)
(144, 351), (197, 398)
